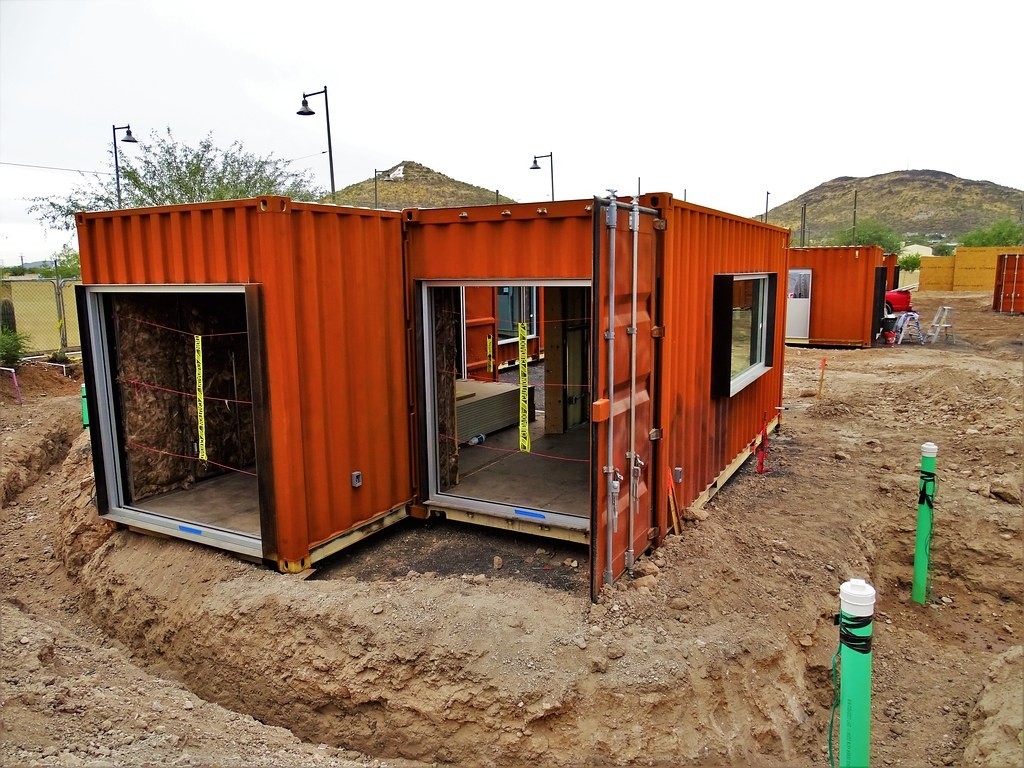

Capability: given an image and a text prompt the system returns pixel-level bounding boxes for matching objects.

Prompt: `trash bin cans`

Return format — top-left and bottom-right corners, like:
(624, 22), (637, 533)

(882, 316), (897, 338)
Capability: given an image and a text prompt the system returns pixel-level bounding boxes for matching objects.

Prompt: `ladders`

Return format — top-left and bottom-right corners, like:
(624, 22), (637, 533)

(923, 305), (957, 346)
(894, 312), (926, 347)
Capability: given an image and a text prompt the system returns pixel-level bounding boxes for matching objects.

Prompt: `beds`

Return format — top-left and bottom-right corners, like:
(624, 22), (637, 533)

(456, 377), (534, 447)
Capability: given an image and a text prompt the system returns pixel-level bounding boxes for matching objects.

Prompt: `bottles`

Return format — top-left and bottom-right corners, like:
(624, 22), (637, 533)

(468, 433), (486, 445)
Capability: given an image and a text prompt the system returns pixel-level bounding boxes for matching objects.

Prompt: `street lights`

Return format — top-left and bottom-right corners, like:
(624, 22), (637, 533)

(373, 169), (393, 210)
(529, 152), (556, 201)
(112, 123), (138, 209)
(296, 84), (338, 205)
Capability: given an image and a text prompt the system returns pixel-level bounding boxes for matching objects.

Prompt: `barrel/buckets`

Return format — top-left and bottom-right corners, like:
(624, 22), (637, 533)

(884, 332), (895, 345)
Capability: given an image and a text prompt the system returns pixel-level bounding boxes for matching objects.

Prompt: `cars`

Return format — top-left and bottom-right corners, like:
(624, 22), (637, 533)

(886, 290), (915, 315)
